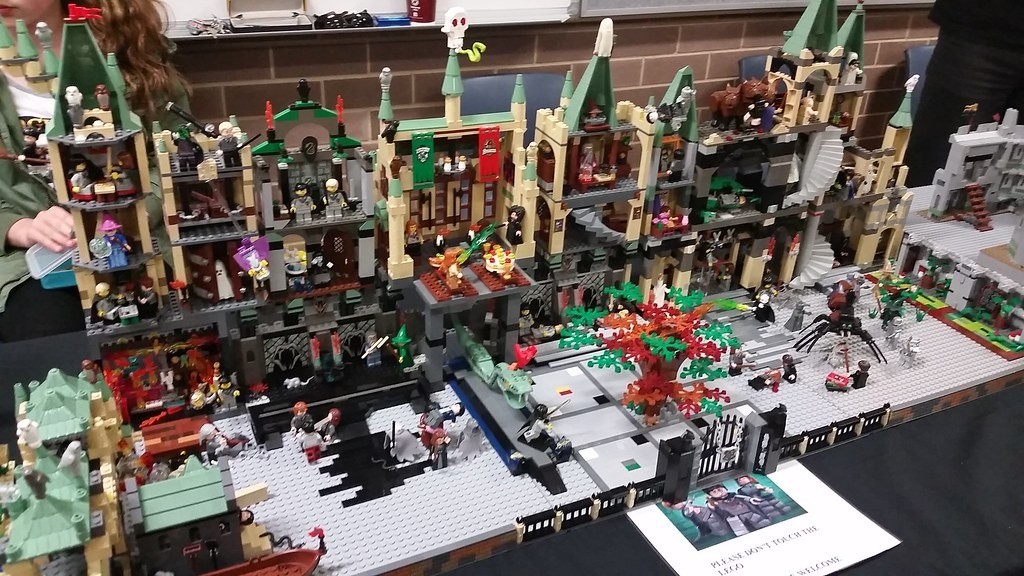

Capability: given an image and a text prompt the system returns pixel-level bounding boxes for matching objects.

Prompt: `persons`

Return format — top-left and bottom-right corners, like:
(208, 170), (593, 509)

(902, 0), (1022, 188)
(1, 0), (193, 345)
